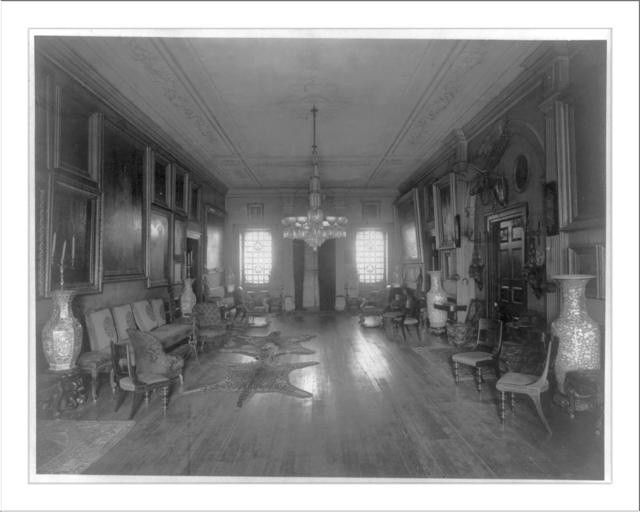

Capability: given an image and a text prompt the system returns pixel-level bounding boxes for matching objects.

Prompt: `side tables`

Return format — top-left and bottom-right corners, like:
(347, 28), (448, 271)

(37, 370), (91, 420)
(434, 303), (466, 321)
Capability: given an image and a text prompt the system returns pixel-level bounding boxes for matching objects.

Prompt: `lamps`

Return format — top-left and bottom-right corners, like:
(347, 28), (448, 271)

(280, 105), (349, 253)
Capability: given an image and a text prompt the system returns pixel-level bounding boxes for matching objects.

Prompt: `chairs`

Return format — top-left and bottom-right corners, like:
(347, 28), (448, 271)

(110, 340), (170, 420)
(357, 284), (395, 328)
(447, 299), (485, 345)
(192, 301), (229, 353)
(233, 286), (256, 322)
(393, 299), (423, 344)
(451, 318), (503, 393)
(495, 336), (559, 436)
(126, 327), (185, 403)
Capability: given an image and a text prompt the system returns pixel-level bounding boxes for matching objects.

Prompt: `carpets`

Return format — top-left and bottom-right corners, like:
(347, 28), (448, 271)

(181, 356), (320, 408)
(37, 419), (137, 474)
(217, 326), (318, 362)
(409, 343), (499, 383)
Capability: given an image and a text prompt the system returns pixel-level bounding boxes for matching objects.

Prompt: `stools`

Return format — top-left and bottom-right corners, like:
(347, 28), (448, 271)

(78, 351), (118, 405)
(564, 368), (604, 434)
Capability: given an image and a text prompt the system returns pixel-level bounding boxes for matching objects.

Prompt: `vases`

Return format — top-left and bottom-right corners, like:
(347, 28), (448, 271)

(550, 274), (602, 400)
(179, 278), (197, 318)
(40, 290), (84, 372)
(425, 270), (449, 330)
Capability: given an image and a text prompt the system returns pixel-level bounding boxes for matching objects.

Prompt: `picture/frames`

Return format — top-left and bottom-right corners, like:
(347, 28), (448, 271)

(35, 61), (201, 298)
(362, 200), (382, 222)
(203, 203), (226, 275)
(246, 203), (264, 222)
(391, 171), (458, 264)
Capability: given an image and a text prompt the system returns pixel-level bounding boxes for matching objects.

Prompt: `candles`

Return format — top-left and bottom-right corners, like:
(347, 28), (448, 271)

(51, 232), (76, 265)
(184, 250), (193, 267)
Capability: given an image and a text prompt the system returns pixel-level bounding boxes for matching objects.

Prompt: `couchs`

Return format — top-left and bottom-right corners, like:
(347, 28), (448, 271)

(83, 298), (193, 375)
